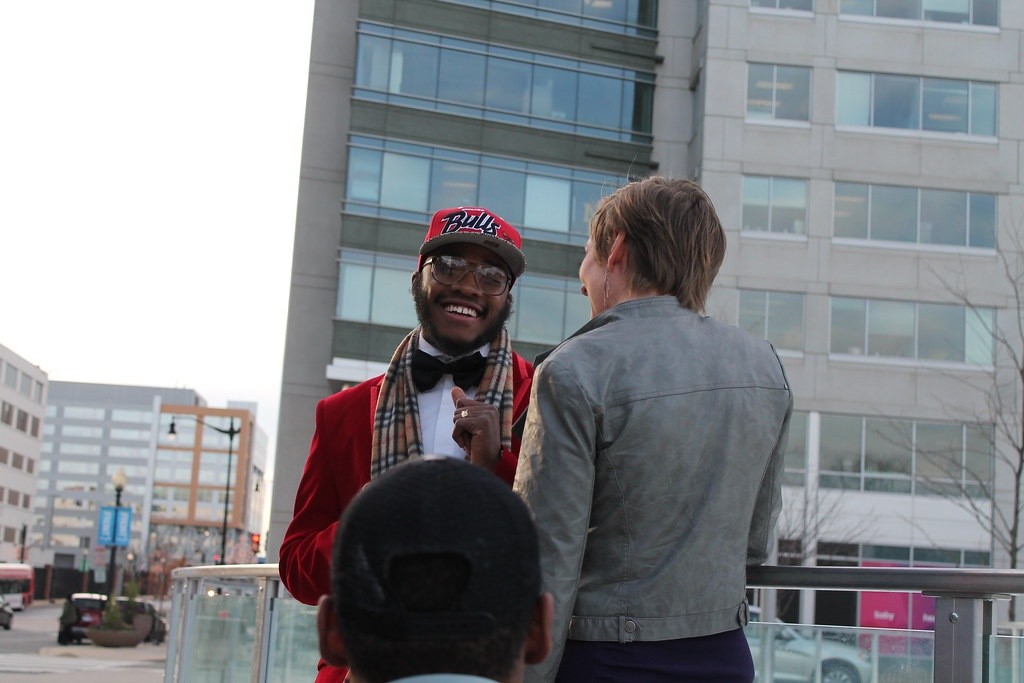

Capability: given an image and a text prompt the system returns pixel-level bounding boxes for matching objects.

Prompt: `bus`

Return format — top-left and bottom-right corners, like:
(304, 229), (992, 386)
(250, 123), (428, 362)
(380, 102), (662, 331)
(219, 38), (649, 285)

(0, 563), (34, 611)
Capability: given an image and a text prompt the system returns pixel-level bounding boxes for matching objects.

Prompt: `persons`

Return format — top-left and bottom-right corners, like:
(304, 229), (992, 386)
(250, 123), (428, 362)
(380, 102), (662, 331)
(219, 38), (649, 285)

(317, 455), (553, 683)
(279, 205), (533, 682)
(62, 593), (77, 643)
(514, 174), (795, 683)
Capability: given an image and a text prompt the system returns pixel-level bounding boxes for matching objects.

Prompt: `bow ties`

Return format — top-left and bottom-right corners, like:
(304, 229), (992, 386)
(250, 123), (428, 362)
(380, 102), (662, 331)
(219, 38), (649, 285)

(410, 348), (487, 393)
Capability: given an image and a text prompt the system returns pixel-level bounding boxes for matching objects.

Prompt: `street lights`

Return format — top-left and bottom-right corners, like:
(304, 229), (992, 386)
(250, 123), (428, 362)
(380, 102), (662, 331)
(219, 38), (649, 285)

(106, 467), (125, 596)
(166, 414), (243, 567)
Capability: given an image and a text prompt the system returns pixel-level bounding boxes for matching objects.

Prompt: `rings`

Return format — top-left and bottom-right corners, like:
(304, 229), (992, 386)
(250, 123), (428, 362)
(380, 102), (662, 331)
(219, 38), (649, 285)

(462, 410), (468, 418)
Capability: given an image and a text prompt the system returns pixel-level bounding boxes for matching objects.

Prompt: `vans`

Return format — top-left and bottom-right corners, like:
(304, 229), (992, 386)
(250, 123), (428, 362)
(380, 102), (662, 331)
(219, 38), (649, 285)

(56, 594), (121, 645)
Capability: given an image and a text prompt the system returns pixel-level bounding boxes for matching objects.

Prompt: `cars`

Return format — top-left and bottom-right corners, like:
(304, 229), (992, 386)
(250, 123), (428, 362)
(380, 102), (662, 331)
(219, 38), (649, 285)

(743, 607), (874, 683)
(0, 596), (14, 629)
(114, 599), (167, 647)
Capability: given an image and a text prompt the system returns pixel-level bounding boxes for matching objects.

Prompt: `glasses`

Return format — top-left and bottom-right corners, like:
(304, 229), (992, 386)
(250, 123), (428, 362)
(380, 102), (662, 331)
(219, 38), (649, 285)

(421, 254), (512, 296)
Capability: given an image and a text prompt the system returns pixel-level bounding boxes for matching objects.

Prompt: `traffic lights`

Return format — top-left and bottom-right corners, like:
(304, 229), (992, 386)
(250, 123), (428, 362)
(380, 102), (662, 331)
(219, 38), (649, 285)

(250, 533), (260, 553)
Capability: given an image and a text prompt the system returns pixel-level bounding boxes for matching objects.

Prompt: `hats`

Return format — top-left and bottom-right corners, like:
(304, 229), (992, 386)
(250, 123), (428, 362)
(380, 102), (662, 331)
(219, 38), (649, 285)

(418, 206), (527, 293)
